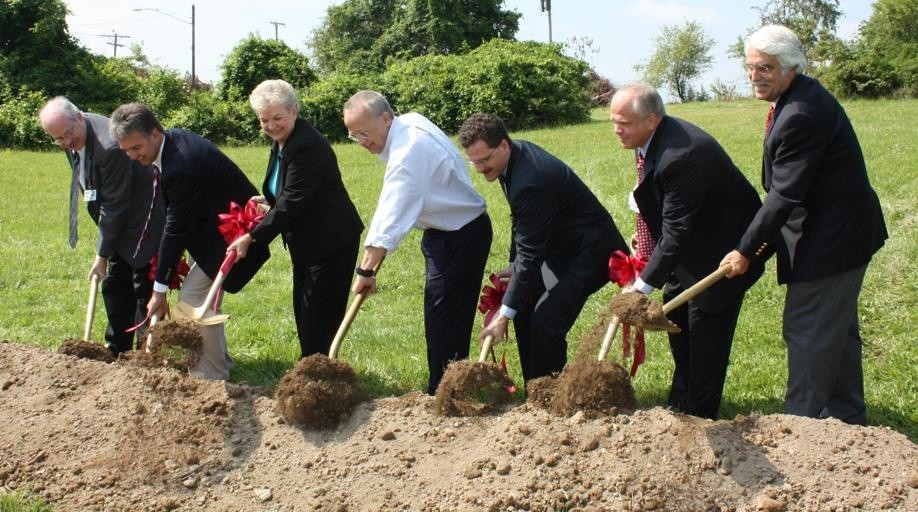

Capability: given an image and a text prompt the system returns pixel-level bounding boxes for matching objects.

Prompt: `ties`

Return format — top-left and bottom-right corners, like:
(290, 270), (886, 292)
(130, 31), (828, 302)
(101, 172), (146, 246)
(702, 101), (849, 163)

(765, 106), (775, 130)
(70, 152), (80, 247)
(132, 165), (159, 257)
(636, 152), (656, 259)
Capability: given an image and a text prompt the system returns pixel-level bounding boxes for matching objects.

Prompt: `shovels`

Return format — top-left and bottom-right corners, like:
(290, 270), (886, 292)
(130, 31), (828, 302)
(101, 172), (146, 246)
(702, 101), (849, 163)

(626, 262), (732, 334)
(171, 249), (236, 328)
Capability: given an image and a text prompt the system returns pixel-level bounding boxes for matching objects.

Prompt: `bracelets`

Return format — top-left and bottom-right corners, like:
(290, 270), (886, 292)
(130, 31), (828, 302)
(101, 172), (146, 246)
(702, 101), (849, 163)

(355, 265), (374, 277)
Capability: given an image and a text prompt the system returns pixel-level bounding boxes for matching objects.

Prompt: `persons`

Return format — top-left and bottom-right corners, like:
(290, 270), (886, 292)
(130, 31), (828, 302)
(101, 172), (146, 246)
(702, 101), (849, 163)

(455, 114), (631, 396)
(343, 89), (493, 395)
(225, 78), (365, 361)
(39, 95), (166, 358)
(719, 23), (888, 427)
(609, 84), (765, 421)
(109, 101), (272, 381)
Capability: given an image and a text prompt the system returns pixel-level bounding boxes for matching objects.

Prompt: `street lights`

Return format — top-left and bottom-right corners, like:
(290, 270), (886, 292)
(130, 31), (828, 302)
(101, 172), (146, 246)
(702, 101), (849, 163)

(133, 3), (197, 87)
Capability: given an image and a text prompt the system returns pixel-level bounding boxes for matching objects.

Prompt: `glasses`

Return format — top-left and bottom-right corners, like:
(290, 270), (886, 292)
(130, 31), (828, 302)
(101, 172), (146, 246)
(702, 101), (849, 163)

(348, 119), (379, 141)
(744, 64), (769, 73)
(49, 122), (76, 146)
(470, 145), (502, 165)
